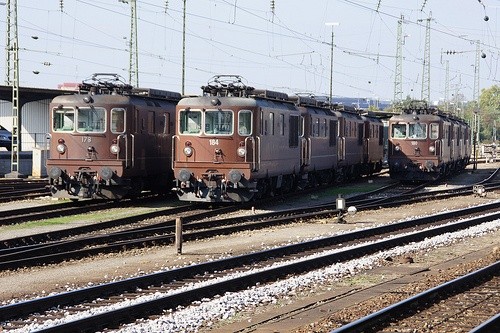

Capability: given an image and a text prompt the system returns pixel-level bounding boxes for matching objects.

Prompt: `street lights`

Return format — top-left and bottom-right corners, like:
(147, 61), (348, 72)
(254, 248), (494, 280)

(472, 105), (479, 169)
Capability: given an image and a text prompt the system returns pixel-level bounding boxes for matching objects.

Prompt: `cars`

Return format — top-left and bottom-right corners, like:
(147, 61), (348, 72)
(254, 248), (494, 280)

(0, 125), (13, 151)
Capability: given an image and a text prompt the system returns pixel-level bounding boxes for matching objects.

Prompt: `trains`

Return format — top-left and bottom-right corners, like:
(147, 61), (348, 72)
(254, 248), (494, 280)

(45, 72), (183, 202)
(170, 74), (397, 208)
(388, 100), (472, 184)
